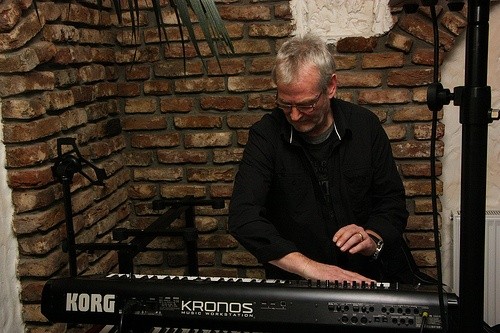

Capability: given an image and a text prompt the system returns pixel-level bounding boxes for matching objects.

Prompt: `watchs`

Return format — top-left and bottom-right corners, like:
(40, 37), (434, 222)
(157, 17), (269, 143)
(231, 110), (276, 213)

(366, 229), (386, 262)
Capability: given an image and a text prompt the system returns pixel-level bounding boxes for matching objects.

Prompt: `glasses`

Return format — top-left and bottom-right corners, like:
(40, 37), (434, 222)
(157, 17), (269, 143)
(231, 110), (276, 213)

(274, 89), (323, 114)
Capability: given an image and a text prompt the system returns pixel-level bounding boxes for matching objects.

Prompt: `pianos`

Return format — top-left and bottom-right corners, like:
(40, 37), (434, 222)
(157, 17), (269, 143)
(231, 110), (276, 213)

(41, 273), (460, 333)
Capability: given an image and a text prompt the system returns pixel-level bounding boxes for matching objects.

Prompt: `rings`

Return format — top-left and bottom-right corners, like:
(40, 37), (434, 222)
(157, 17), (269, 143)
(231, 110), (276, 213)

(356, 233), (364, 242)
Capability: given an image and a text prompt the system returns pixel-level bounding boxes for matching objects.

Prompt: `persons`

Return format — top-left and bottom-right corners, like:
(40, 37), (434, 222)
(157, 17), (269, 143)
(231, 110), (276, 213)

(228, 33), (440, 285)
